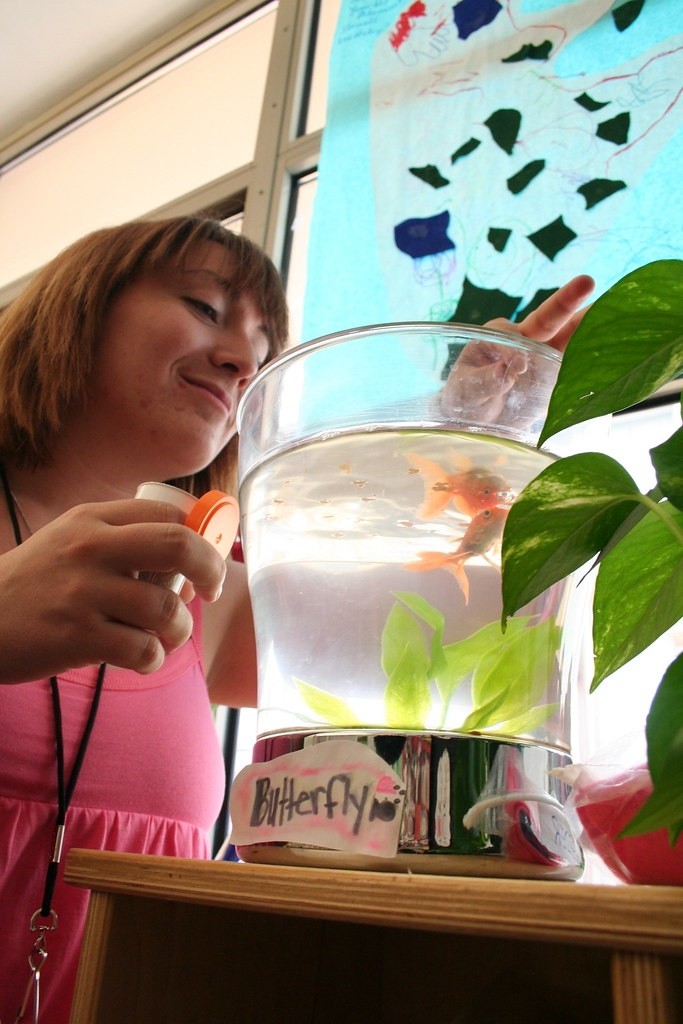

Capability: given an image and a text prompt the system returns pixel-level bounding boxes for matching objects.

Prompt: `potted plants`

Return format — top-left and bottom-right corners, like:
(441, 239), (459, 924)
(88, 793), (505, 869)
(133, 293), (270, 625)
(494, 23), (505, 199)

(502, 258), (683, 889)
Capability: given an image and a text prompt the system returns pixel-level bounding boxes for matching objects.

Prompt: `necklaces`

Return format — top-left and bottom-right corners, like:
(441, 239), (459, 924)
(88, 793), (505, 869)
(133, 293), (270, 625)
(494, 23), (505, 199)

(7, 482), (49, 549)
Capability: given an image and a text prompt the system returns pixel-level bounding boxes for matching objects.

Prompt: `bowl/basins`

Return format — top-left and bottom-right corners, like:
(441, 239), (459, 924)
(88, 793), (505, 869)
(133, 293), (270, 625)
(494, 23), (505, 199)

(576, 762), (683, 888)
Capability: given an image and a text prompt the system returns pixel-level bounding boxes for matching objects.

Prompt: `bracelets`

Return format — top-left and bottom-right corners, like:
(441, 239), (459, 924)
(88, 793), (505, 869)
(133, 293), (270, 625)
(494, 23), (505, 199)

(435, 388), (448, 419)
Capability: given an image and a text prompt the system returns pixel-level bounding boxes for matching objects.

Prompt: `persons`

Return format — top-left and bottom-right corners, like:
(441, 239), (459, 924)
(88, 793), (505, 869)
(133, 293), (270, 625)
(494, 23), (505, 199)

(0, 215), (611, 1024)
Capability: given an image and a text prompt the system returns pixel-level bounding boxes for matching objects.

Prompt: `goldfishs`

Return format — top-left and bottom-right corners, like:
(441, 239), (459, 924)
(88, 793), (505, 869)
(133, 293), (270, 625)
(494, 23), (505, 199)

(404, 447), (512, 606)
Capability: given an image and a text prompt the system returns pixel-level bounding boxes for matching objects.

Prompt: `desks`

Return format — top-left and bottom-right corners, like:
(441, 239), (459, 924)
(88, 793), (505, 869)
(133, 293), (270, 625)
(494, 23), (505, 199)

(64, 849), (683, 1023)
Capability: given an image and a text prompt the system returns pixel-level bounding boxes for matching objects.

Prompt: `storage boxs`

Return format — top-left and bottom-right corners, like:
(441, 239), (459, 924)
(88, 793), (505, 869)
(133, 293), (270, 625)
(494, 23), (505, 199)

(234, 321), (612, 883)
